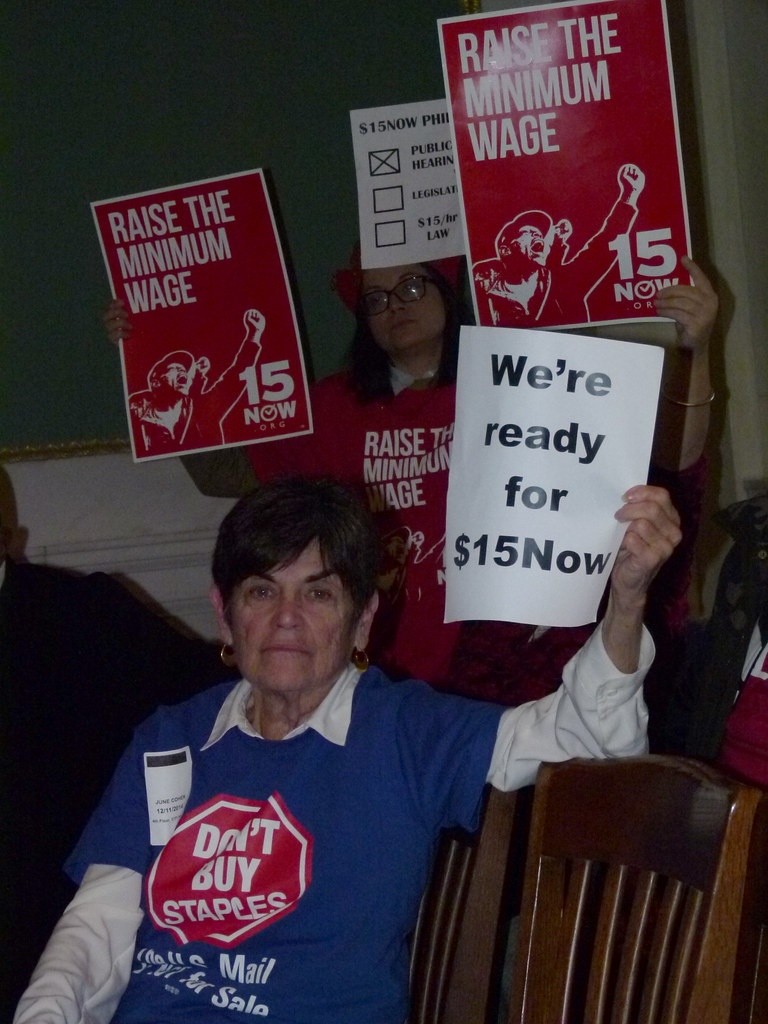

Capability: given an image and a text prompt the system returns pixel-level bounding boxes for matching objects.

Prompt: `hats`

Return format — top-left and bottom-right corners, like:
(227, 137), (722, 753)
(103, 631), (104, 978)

(333, 241), (464, 320)
(494, 210), (554, 259)
(147, 351), (195, 391)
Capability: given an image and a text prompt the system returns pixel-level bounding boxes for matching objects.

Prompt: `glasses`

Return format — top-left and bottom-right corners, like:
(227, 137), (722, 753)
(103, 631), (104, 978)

(356, 275), (437, 317)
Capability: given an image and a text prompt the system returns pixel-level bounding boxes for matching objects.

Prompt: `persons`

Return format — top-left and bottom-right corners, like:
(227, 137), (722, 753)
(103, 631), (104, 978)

(0, 464), (247, 1024)
(657, 494), (768, 796)
(11, 477), (683, 1024)
(103, 233), (720, 706)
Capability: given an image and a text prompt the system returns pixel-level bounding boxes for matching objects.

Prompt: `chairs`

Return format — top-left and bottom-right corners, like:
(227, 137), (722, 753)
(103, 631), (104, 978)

(507, 754), (762, 1024)
(408, 785), (518, 1024)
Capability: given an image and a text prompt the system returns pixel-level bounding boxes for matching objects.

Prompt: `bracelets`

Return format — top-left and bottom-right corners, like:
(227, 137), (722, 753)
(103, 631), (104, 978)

(661, 381), (715, 407)
